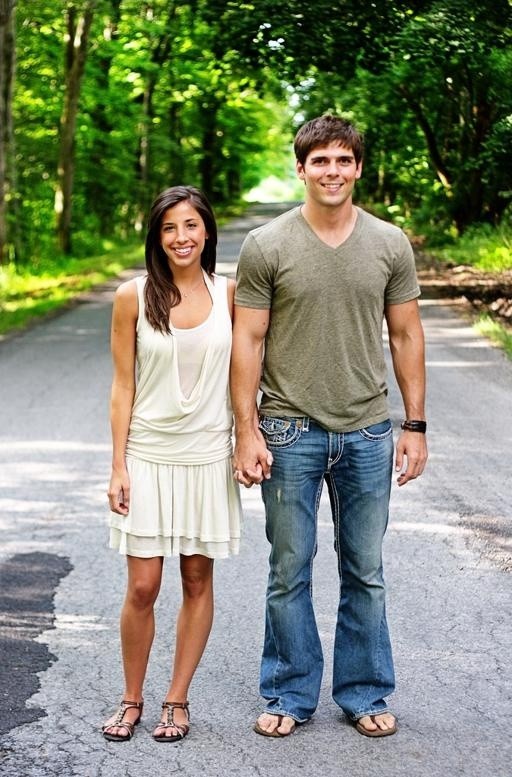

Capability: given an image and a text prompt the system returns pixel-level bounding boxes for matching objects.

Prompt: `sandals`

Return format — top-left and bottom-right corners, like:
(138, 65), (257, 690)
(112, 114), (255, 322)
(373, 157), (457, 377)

(102, 700), (145, 742)
(154, 702), (191, 742)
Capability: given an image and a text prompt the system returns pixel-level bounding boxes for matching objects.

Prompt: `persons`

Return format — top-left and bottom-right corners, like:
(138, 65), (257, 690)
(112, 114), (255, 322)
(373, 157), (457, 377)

(231, 113), (429, 740)
(100, 180), (271, 744)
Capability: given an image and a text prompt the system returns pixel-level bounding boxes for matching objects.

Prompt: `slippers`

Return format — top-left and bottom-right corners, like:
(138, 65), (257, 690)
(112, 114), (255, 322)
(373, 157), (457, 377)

(354, 712), (397, 736)
(255, 715), (297, 737)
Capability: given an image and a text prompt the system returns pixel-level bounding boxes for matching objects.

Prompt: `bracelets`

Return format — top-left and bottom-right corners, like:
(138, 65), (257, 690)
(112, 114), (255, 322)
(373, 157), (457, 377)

(399, 420), (427, 434)
